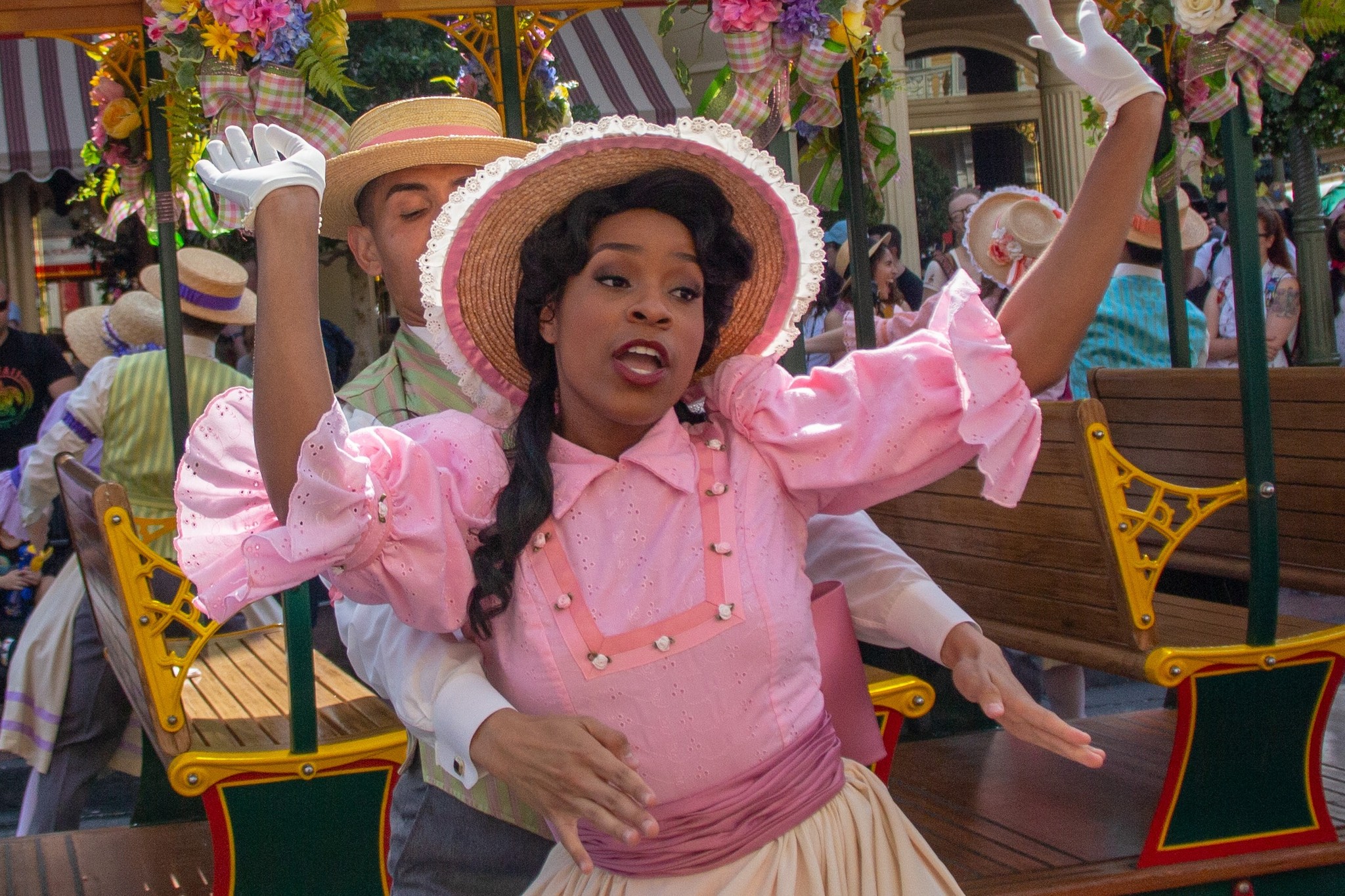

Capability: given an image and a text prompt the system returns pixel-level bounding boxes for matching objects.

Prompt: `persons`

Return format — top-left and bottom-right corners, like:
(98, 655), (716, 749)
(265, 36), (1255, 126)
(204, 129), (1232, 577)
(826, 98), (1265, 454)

(315, 95), (1106, 896)
(0, 248), (355, 836)
(173, 0), (1166, 896)
(801, 176), (1345, 720)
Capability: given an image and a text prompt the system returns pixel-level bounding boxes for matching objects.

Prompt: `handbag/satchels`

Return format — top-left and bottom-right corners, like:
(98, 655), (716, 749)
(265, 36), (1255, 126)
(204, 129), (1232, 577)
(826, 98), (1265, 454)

(813, 578), (889, 766)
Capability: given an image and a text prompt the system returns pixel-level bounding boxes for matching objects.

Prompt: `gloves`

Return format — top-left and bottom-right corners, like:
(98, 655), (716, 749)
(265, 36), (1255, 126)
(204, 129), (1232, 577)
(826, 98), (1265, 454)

(194, 121), (327, 235)
(1016, 0), (1166, 126)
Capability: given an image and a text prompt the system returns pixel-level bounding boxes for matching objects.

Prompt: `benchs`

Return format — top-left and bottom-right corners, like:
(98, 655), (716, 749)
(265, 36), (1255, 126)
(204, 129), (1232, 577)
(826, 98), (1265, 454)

(863, 365), (1345, 896)
(54, 452), (410, 896)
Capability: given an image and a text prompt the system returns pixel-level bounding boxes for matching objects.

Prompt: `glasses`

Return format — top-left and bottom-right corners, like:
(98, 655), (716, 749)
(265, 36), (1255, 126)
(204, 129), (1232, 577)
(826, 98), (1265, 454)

(1194, 205), (1209, 214)
(950, 202), (978, 223)
(1214, 202), (1228, 212)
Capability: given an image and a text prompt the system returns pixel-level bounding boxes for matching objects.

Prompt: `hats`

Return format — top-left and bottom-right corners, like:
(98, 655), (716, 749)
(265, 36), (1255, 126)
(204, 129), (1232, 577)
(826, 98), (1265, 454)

(963, 185), (1070, 291)
(822, 219), (848, 245)
(1179, 182), (1208, 204)
(138, 246), (258, 327)
(61, 291), (166, 370)
(1124, 176), (1210, 253)
(316, 96), (548, 245)
(420, 113), (826, 424)
(7, 301), (21, 324)
(833, 232), (892, 296)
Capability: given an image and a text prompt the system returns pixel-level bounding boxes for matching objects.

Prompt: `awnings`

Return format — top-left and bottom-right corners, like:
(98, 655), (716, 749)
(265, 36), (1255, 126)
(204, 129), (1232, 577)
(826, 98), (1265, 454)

(539, 7), (693, 127)
(0, 34), (109, 182)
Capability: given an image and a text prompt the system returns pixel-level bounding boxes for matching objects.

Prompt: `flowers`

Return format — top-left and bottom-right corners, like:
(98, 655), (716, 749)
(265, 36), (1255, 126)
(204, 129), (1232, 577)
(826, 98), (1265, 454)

(68, 0), (375, 250)
(1081, 0), (1345, 217)
(430, 10), (579, 144)
(659, 0), (902, 224)
